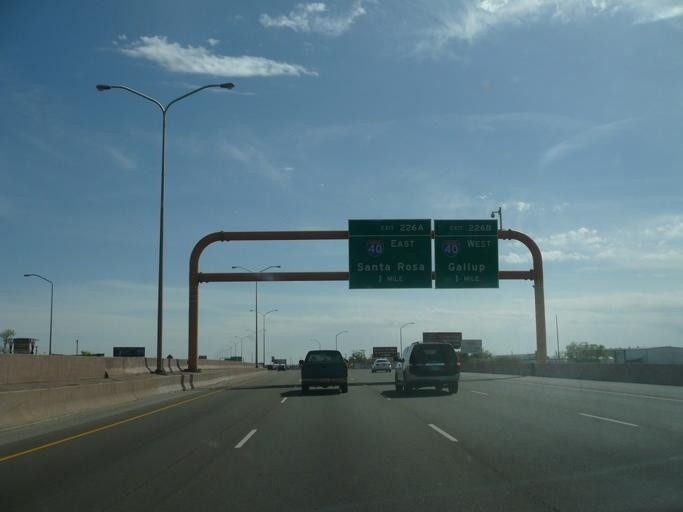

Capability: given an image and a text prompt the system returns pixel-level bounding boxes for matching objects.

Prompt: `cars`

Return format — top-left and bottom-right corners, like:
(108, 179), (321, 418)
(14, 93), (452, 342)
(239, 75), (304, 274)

(299, 350), (349, 393)
(371, 359), (392, 373)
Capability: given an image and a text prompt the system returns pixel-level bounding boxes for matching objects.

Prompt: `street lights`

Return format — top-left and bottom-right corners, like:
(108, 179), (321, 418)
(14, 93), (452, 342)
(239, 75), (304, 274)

(23, 273), (53, 355)
(94, 81), (236, 374)
(248, 308), (278, 368)
(231, 265), (281, 368)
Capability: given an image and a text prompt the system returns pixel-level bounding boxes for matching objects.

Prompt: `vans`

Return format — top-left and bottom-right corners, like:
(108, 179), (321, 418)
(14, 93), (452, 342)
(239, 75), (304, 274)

(394, 342), (461, 393)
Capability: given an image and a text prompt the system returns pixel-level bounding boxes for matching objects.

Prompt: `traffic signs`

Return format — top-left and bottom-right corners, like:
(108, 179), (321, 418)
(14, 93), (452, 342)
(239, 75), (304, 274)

(348, 218), (432, 288)
(436, 220), (499, 288)
